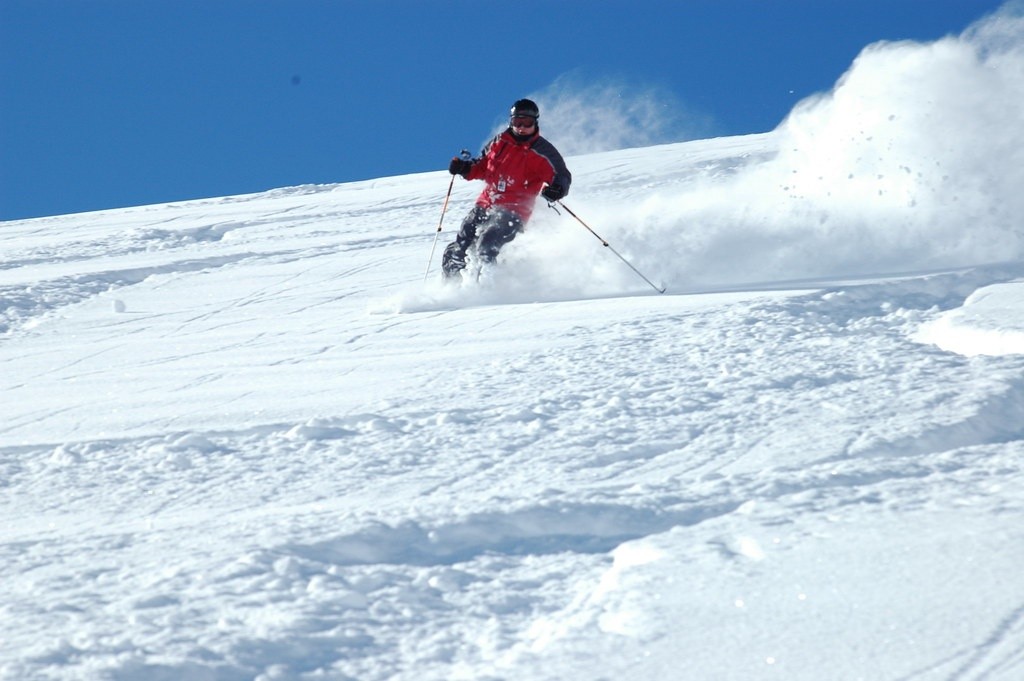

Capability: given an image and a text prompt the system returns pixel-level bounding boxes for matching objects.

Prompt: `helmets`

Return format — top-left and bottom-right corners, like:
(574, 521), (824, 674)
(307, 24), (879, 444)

(511, 99), (540, 118)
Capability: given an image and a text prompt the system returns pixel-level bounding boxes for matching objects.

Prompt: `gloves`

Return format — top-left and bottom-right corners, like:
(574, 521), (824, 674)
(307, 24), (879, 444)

(541, 184), (564, 202)
(449, 157), (472, 175)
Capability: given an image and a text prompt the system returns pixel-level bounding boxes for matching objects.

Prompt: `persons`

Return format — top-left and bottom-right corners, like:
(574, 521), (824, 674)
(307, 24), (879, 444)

(440, 97), (571, 281)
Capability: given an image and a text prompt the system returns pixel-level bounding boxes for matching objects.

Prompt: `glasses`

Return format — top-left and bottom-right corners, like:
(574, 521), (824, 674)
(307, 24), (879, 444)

(510, 115), (535, 128)
